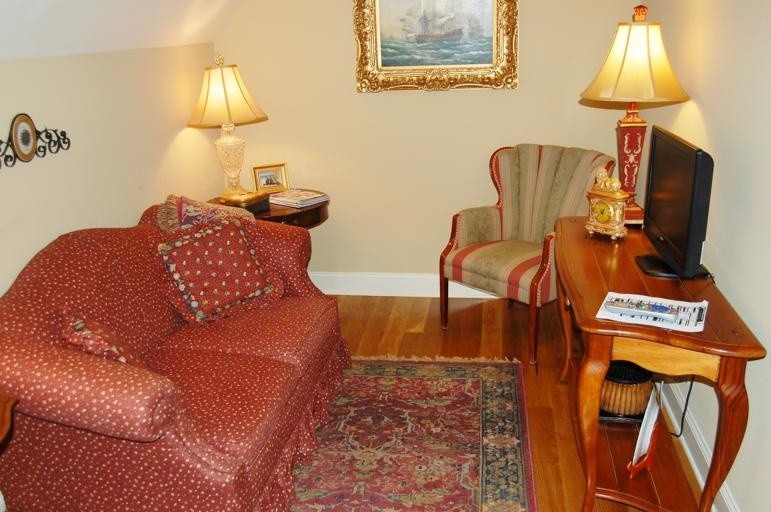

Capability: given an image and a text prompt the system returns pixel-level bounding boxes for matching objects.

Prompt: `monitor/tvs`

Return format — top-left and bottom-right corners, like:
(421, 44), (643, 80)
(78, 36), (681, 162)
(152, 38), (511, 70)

(634, 124), (715, 278)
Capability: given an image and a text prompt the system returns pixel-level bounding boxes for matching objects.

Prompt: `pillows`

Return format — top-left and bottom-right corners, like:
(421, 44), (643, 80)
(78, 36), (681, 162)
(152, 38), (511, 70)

(58, 305), (152, 374)
(153, 195), (287, 328)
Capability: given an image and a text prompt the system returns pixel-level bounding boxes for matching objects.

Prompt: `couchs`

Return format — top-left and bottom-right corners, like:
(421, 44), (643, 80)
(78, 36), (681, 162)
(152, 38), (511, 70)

(0, 199), (347, 510)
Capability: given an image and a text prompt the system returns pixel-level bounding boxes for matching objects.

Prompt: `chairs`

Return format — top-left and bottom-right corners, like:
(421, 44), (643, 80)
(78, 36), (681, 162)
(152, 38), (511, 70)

(436, 143), (613, 369)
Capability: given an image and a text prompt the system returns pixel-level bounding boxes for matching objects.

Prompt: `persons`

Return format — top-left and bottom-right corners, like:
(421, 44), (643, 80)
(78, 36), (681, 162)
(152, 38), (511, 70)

(264, 176), (280, 185)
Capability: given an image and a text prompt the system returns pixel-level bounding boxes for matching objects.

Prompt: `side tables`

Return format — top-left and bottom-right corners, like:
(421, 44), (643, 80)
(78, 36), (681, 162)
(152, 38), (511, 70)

(206, 188), (329, 231)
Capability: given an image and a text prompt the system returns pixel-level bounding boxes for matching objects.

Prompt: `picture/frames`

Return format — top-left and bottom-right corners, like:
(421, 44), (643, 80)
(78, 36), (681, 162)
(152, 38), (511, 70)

(249, 160), (290, 197)
(352, 1), (521, 91)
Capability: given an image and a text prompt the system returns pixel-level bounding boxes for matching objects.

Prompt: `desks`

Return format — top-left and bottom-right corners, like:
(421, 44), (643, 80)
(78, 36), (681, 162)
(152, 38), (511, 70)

(553, 215), (766, 510)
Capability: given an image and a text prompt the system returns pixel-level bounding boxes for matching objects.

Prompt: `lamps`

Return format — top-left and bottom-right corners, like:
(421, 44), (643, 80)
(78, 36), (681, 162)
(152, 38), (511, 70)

(186, 54), (271, 216)
(579, 6), (694, 225)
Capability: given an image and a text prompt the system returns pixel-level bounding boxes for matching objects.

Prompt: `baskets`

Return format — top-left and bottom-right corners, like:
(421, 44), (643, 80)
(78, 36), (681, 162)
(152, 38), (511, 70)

(601, 378), (654, 416)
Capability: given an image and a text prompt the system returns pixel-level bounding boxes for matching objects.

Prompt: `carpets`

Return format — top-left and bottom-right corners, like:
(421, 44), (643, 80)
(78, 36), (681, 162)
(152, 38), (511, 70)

(281, 354), (535, 512)
